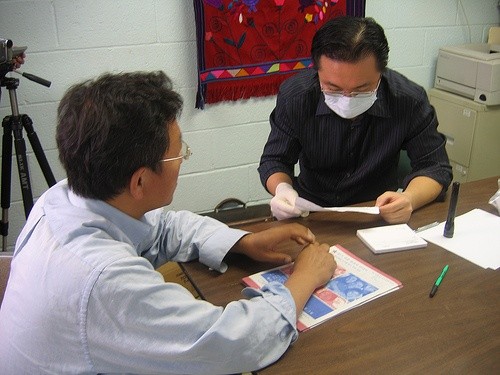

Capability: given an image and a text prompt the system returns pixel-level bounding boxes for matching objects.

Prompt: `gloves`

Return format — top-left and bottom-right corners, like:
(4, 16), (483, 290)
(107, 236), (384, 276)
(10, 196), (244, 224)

(270, 183), (301, 221)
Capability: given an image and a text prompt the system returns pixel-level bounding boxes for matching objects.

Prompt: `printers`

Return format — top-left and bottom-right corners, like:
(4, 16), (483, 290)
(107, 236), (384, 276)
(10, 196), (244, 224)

(434, 28), (500, 106)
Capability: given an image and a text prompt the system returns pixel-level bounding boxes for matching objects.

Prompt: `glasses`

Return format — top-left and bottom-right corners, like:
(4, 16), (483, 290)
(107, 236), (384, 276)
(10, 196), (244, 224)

(158, 141), (191, 163)
(320, 79), (381, 98)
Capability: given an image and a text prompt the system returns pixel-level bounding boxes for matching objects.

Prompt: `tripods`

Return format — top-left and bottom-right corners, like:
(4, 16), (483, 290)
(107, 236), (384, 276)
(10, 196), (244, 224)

(0, 63), (57, 253)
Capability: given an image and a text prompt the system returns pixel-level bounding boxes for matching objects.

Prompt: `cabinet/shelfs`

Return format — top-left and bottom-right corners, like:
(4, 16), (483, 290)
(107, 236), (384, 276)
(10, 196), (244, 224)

(427, 88), (500, 184)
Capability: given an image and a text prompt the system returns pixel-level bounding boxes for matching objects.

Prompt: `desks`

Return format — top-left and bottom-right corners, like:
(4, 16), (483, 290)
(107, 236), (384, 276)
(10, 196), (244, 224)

(178, 175), (500, 375)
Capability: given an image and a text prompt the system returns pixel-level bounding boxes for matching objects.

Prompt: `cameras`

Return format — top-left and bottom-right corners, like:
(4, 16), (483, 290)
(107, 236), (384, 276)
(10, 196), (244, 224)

(0, 38), (27, 63)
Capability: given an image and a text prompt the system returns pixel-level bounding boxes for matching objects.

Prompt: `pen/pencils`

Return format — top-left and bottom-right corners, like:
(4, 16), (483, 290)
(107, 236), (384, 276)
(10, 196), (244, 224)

(429, 264), (450, 297)
(414, 221), (438, 231)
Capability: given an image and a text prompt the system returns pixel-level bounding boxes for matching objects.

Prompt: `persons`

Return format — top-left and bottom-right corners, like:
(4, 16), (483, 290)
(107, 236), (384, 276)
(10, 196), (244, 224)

(12, 51), (25, 70)
(0, 72), (336, 375)
(258, 16), (453, 225)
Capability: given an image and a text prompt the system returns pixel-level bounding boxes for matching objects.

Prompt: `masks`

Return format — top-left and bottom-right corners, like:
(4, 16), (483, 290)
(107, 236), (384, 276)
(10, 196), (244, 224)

(324, 91), (377, 120)
